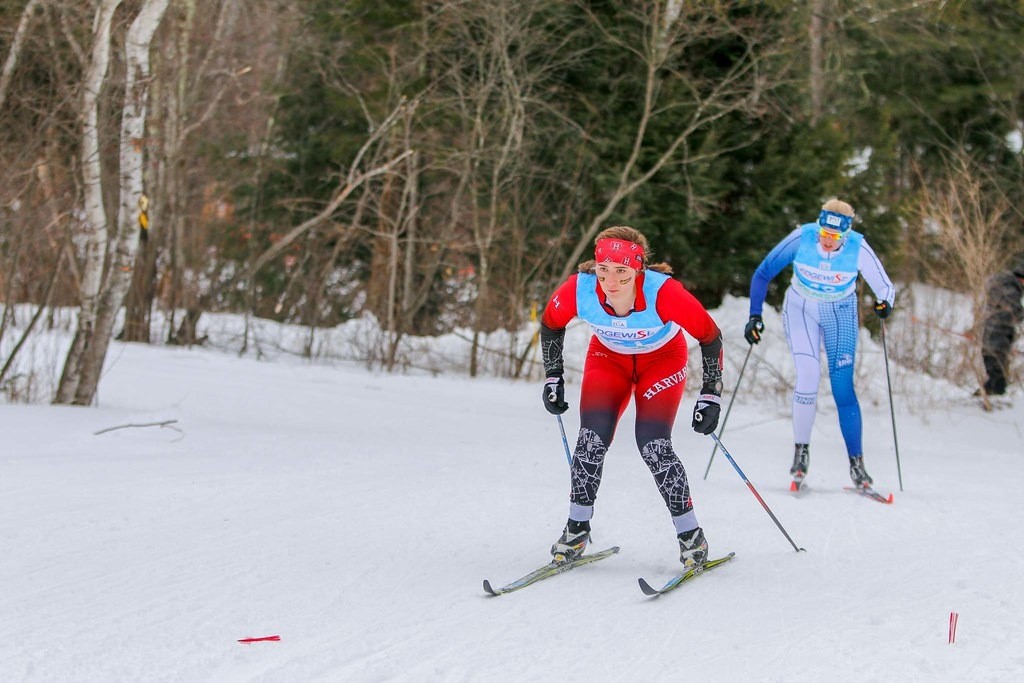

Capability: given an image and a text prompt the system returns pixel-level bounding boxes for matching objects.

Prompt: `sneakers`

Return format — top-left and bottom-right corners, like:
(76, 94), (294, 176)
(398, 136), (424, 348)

(849, 456), (872, 484)
(551, 529), (589, 562)
(678, 528), (709, 567)
(790, 443), (811, 474)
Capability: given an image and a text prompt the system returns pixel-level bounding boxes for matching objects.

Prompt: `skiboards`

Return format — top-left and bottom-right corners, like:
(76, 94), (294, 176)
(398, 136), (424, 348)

(482, 545), (738, 597)
(789, 485), (894, 505)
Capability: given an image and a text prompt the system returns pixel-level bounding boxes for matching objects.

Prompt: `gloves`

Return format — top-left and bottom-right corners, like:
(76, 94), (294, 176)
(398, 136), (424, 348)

(692, 381), (723, 435)
(874, 299), (891, 318)
(542, 369), (569, 414)
(744, 316), (765, 344)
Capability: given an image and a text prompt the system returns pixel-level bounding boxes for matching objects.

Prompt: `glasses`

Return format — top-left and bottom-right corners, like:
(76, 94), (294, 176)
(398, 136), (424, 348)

(819, 224), (850, 241)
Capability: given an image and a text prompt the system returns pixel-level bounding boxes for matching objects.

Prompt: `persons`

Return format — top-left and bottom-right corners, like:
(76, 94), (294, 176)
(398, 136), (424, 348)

(744, 200), (895, 485)
(541, 228), (724, 569)
